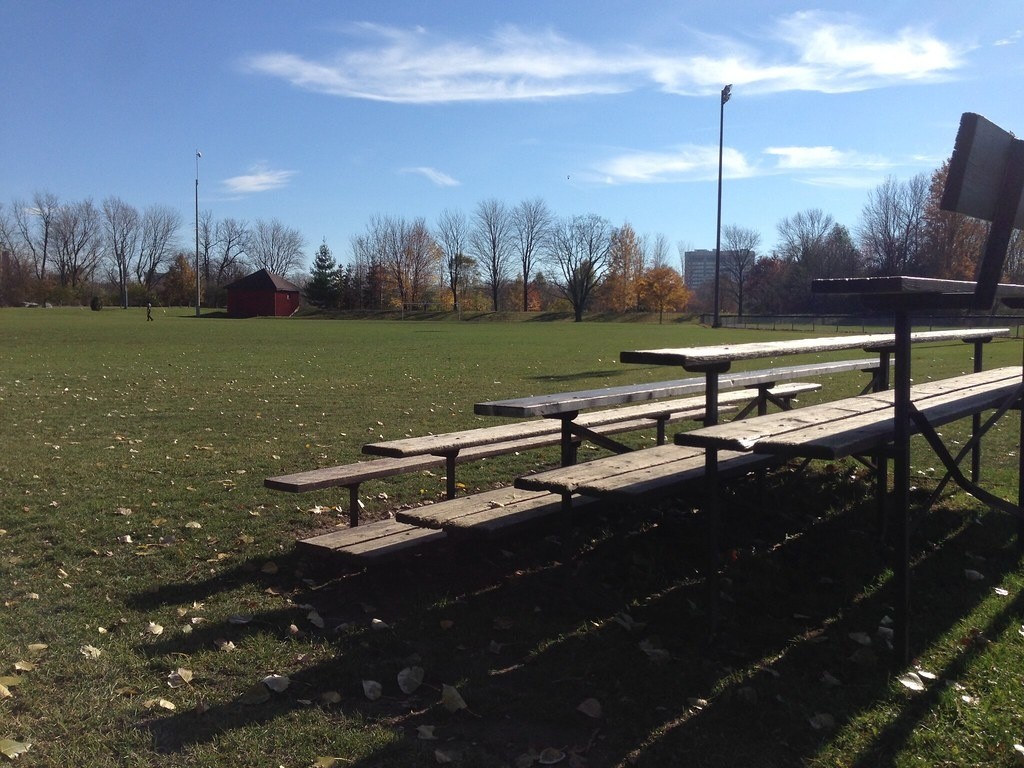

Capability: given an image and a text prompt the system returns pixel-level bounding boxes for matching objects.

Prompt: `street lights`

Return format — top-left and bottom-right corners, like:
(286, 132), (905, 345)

(711, 82), (734, 331)
(195, 147), (202, 319)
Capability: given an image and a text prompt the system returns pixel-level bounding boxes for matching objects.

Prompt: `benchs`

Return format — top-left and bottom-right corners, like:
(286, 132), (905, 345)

(263, 111), (1023, 673)
